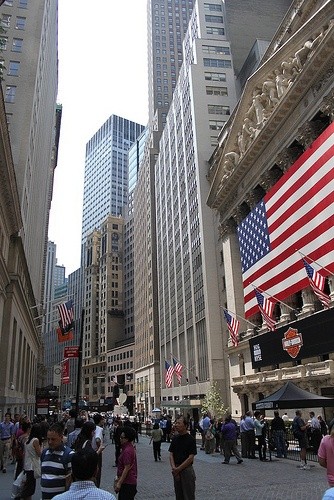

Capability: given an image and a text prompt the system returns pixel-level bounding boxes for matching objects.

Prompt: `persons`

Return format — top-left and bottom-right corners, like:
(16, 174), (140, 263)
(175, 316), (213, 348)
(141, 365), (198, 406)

(218, 33), (324, 187)
(0, 408), (334, 500)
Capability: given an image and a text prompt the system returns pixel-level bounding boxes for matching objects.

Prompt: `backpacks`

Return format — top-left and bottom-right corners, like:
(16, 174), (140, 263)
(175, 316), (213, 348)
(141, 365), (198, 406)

(13, 434), (30, 457)
(70, 440), (91, 477)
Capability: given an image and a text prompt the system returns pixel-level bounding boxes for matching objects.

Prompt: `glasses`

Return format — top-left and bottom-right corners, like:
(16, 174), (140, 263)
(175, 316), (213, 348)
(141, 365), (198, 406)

(119, 436), (126, 440)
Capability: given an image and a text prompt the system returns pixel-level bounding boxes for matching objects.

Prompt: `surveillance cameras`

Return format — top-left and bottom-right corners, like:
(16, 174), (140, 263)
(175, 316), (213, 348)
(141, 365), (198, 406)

(11, 385), (14, 390)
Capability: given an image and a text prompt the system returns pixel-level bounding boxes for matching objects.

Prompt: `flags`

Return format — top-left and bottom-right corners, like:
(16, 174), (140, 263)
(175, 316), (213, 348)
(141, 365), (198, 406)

(236, 122), (334, 320)
(164, 361), (174, 387)
(57, 299), (75, 342)
(254, 288), (276, 332)
(223, 309), (240, 348)
(301, 257), (330, 309)
(173, 357), (183, 384)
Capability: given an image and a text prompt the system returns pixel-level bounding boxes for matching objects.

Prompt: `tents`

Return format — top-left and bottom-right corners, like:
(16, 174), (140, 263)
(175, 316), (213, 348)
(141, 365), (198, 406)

(251, 380), (334, 425)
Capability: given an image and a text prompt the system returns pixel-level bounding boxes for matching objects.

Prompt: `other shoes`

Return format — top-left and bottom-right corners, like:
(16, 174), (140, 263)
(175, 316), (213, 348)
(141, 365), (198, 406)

(158, 456), (161, 461)
(200, 446), (287, 464)
(155, 459), (157, 463)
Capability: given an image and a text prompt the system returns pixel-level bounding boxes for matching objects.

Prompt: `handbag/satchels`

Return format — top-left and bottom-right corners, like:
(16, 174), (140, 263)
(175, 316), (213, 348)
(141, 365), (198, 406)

(34, 457), (41, 479)
(10, 470), (26, 500)
